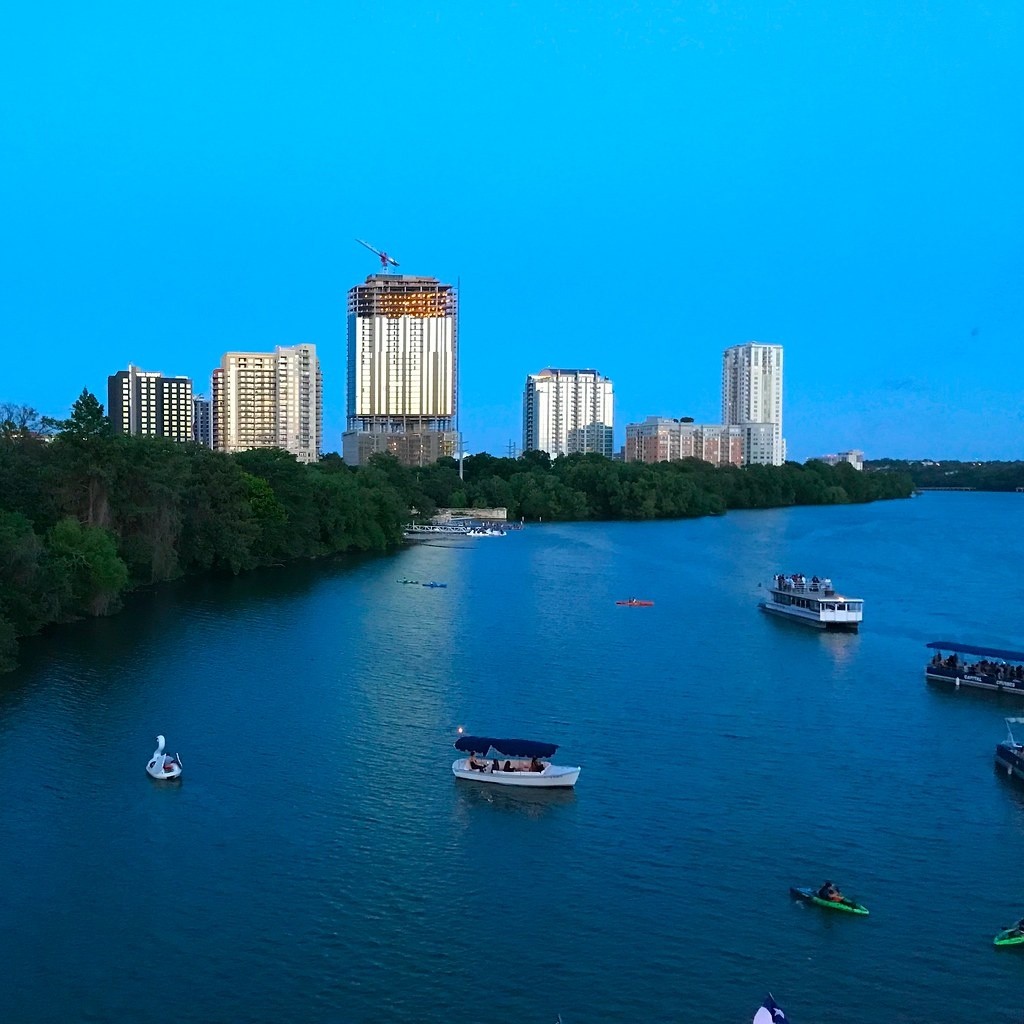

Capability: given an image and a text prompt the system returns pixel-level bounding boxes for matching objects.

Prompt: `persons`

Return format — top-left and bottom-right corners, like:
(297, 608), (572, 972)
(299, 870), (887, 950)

(470, 752), (489, 773)
(490, 759), (499, 773)
(529, 756), (545, 772)
(932, 652), (1024, 682)
(819, 882), (839, 901)
(773, 572), (832, 591)
(163, 752), (175, 772)
(502, 761), (516, 772)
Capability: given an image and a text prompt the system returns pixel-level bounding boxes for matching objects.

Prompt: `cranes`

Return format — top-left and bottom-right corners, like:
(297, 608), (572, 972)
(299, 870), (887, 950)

(355, 238), (400, 273)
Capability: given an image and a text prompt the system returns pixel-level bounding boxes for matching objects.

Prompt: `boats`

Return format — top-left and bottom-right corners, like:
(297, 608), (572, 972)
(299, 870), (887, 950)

(756, 578), (863, 630)
(993, 926), (1024, 946)
(422, 581), (447, 588)
(617, 601), (652, 606)
(791, 886), (870, 916)
(469, 524), (520, 537)
(753, 991), (788, 1024)
(926, 641), (1024, 696)
(147, 735), (183, 780)
(396, 579), (418, 584)
(995, 718), (1024, 782)
(452, 736), (581, 788)
